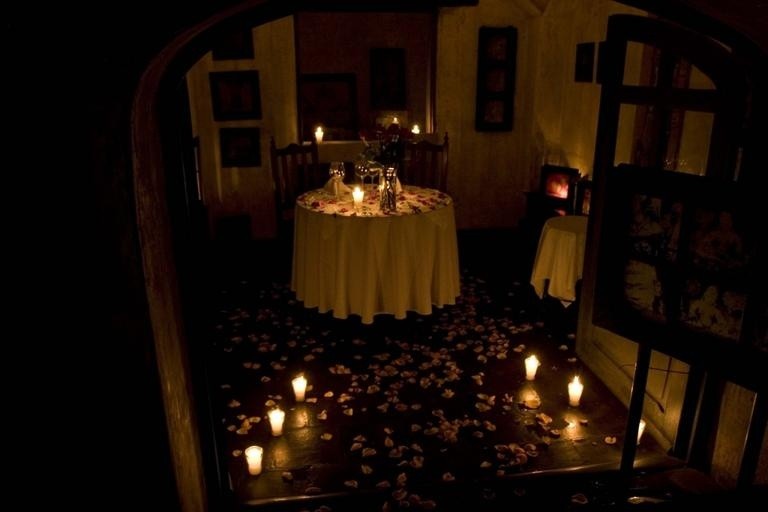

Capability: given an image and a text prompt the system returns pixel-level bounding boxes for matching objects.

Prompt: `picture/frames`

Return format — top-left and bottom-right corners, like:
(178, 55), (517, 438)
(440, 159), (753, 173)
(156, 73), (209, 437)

(208, 28), (262, 168)
(574, 42), (607, 85)
(299, 72), (360, 141)
(474, 24), (518, 132)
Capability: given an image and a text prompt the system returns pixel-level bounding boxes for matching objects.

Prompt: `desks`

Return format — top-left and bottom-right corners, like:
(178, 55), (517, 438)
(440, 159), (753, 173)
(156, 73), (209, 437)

(530, 214), (588, 336)
(289, 184), (463, 352)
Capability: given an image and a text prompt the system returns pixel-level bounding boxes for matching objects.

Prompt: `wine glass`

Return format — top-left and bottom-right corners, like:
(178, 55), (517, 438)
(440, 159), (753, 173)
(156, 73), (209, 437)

(327, 160), (346, 201)
(353, 158), (379, 197)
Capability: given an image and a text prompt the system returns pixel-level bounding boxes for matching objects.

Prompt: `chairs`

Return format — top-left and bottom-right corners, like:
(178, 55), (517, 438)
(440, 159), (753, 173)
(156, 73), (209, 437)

(269, 131), (450, 265)
